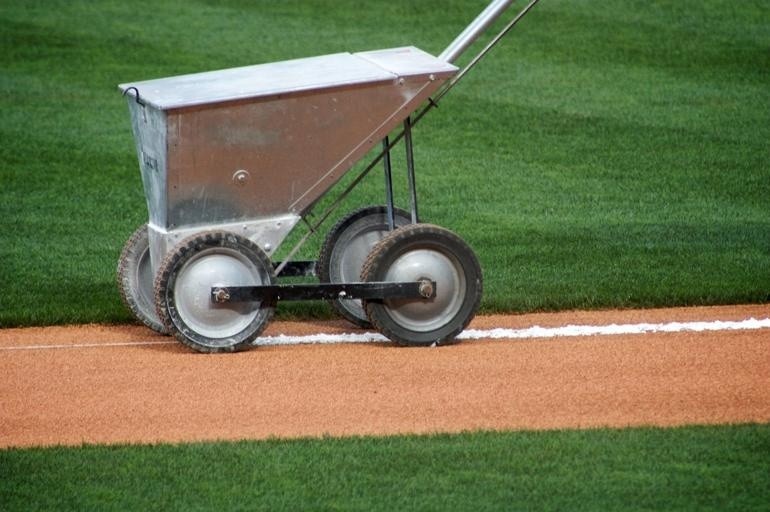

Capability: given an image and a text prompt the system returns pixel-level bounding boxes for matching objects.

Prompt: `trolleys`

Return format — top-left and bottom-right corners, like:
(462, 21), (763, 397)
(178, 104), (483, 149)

(112, 1), (543, 357)
